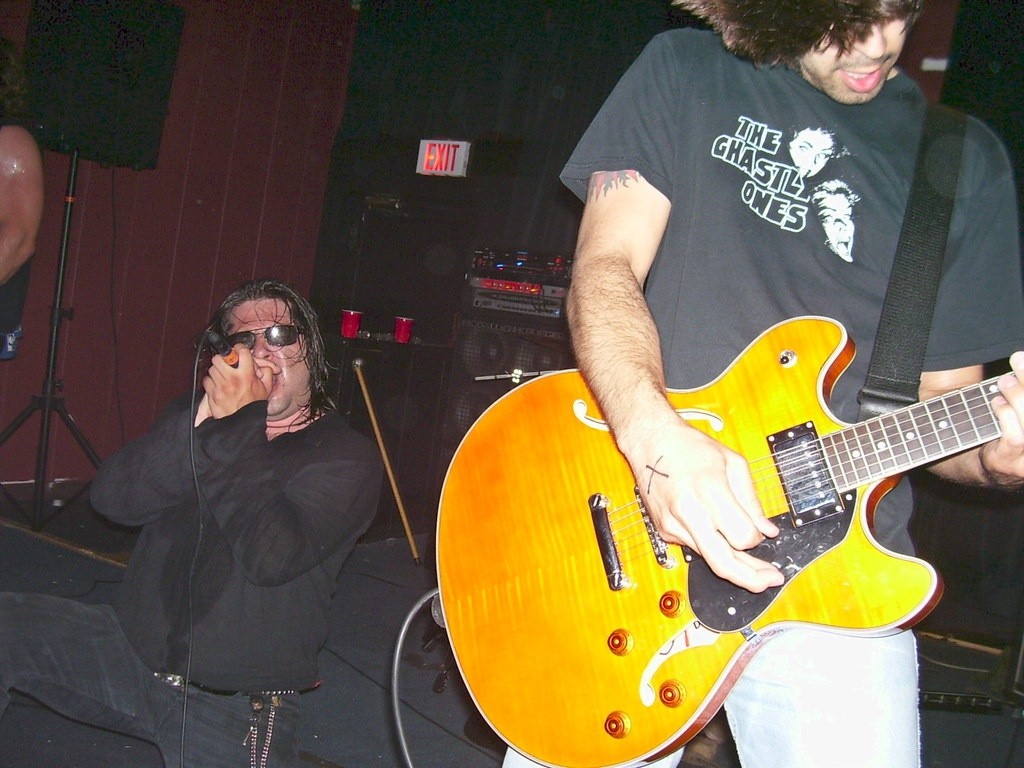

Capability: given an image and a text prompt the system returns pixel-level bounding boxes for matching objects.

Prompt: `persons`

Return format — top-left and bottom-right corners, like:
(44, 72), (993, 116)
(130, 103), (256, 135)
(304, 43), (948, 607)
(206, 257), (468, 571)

(1, 276), (381, 766)
(0, 37), (47, 360)
(496, 1), (1024, 768)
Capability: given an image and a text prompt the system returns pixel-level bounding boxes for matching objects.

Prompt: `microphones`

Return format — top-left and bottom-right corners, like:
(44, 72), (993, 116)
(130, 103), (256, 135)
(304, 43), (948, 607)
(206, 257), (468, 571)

(208, 329), (240, 370)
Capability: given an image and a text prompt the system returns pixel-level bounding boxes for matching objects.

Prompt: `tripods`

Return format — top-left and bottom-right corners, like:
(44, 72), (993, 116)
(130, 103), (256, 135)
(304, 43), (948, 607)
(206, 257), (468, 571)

(0, 150), (107, 533)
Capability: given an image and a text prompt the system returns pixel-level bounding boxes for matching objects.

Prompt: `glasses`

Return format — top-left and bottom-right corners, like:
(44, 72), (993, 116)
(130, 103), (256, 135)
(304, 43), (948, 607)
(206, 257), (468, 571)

(226, 325), (301, 348)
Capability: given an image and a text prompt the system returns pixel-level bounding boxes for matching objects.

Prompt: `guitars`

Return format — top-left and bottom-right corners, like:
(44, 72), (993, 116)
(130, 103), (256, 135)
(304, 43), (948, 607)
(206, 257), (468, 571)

(432, 314), (1024, 768)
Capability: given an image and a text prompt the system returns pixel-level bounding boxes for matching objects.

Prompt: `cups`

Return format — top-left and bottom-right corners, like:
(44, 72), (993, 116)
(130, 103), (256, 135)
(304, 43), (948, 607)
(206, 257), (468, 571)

(341, 310), (362, 339)
(395, 317), (414, 344)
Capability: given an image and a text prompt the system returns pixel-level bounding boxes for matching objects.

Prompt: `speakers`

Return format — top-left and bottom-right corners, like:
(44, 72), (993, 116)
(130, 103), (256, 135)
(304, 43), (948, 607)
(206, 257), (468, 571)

(334, 340), (449, 542)
(901, 464), (1024, 651)
(423, 304), (582, 571)
(23, 0), (188, 170)
